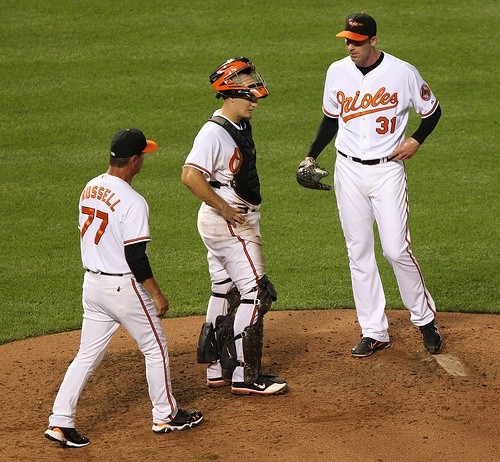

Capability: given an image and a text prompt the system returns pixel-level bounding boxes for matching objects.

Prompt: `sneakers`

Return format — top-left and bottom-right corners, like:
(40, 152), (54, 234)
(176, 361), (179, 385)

(230, 378), (287, 395)
(419, 320), (443, 355)
(152, 409), (203, 433)
(205, 375), (232, 386)
(44, 427), (90, 447)
(351, 337), (391, 357)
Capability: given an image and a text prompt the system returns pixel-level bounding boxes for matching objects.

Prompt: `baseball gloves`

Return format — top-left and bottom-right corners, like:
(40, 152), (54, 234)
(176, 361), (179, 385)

(296, 159), (332, 191)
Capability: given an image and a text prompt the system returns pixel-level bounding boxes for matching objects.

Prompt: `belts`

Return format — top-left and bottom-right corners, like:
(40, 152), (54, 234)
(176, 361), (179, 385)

(337, 150), (393, 166)
(86, 268), (124, 277)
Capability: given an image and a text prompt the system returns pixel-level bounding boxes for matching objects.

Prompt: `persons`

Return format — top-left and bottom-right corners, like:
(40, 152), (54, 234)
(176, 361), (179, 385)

(179, 56), (289, 396)
(295, 13), (445, 357)
(44, 127), (205, 447)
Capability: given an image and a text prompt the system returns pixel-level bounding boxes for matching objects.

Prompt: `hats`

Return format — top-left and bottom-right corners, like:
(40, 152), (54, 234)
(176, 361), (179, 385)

(109, 128), (159, 159)
(335, 11), (377, 42)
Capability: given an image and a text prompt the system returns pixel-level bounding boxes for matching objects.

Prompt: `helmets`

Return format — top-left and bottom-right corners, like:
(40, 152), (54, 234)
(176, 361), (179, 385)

(209, 55), (270, 100)
